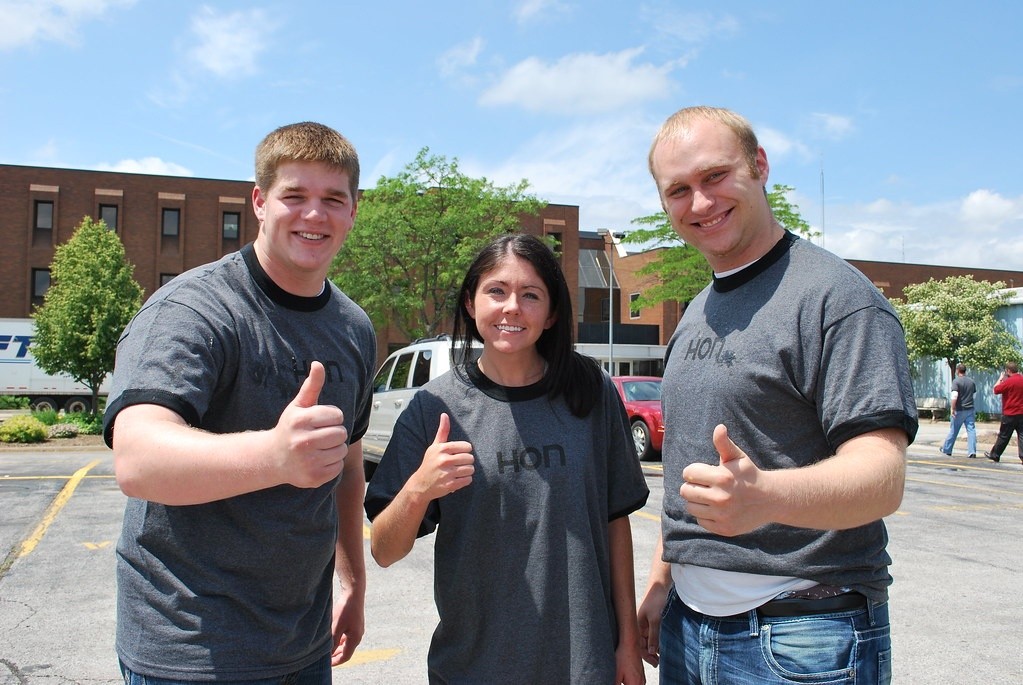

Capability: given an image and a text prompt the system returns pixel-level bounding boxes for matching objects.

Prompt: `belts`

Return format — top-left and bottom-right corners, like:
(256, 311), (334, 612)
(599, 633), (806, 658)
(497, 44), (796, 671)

(733, 591), (879, 618)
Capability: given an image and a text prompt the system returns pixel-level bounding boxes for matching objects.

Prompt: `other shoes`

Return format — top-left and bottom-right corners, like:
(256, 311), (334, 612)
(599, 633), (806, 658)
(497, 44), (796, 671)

(984, 451), (1001, 462)
(966, 454), (976, 458)
(940, 446), (952, 457)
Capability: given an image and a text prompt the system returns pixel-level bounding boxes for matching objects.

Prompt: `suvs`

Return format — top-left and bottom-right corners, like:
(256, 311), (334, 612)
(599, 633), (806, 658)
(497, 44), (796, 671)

(361, 333), (485, 485)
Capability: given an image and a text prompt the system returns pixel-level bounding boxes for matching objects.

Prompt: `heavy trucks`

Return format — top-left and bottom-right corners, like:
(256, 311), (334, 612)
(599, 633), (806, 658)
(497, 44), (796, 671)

(0, 317), (114, 421)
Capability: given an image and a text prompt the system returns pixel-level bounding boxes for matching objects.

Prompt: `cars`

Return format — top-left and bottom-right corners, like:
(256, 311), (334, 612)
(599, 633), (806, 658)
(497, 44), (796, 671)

(609, 374), (668, 462)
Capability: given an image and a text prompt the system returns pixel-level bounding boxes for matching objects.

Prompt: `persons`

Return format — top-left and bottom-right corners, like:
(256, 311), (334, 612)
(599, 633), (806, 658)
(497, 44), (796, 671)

(984, 362), (1023, 465)
(365, 235), (647, 685)
(103, 122), (378, 685)
(637, 107), (918, 685)
(939, 365), (976, 459)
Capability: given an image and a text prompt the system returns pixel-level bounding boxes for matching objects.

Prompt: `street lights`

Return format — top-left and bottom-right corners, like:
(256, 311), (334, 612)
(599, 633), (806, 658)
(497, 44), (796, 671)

(596, 229), (628, 376)
